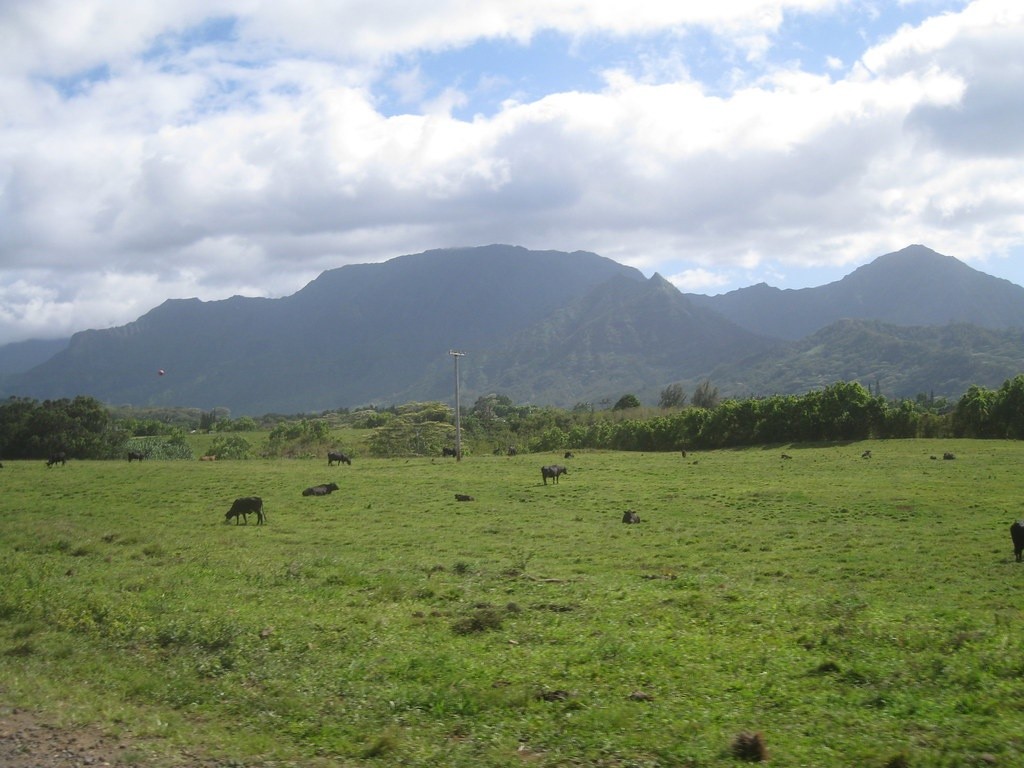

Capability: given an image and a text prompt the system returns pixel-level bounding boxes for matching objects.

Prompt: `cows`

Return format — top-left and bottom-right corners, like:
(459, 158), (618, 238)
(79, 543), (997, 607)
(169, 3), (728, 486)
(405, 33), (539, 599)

(128, 451), (147, 462)
(781, 454), (792, 460)
(442, 445), (458, 457)
(623, 510), (643, 523)
(45, 451), (67, 467)
(328, 451), (352, 467)
(681, 449), (687, 457)
(225, 496), (266, 525)
(507, 447), (516, 455)
(540, 464), (568, 484)
(199, 455), (216, 462)
(563, 451), (575, 458)
(301, 482), (339, 496)
(862, 450), (872, 458)
(455, 494), (475, 501)
